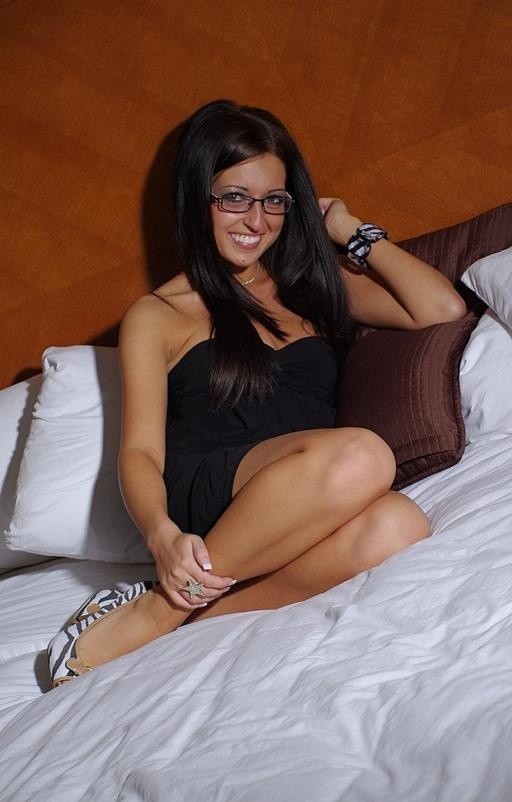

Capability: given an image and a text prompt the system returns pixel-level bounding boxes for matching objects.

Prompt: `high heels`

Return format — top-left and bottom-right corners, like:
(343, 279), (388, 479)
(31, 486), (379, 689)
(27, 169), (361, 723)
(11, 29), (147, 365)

(46, 581), (163, 689)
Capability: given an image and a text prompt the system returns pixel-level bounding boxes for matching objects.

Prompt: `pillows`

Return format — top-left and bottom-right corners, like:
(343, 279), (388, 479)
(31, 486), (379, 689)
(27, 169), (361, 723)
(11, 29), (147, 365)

(0, 206), (510, 568)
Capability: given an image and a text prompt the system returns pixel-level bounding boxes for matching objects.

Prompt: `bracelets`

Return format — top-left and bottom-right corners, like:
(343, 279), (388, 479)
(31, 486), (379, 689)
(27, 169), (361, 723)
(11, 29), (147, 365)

(345, 222), (388, 273)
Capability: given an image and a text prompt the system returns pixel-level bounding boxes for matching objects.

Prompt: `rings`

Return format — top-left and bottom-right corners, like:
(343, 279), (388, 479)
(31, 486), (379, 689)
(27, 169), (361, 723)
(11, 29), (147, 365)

(181, 578), (206, 600)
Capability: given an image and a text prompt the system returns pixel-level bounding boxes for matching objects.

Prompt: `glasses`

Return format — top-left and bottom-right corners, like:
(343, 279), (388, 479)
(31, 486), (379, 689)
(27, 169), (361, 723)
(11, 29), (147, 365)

(211, 192), (295, 215)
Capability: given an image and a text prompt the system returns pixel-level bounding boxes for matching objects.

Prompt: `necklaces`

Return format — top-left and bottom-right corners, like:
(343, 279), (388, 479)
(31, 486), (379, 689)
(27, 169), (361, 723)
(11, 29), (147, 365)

(239, 259), (260, 288)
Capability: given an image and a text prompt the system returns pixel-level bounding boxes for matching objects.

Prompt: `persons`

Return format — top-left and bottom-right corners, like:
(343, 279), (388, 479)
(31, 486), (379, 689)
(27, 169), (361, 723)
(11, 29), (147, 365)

(47, 98), (468, 688)
